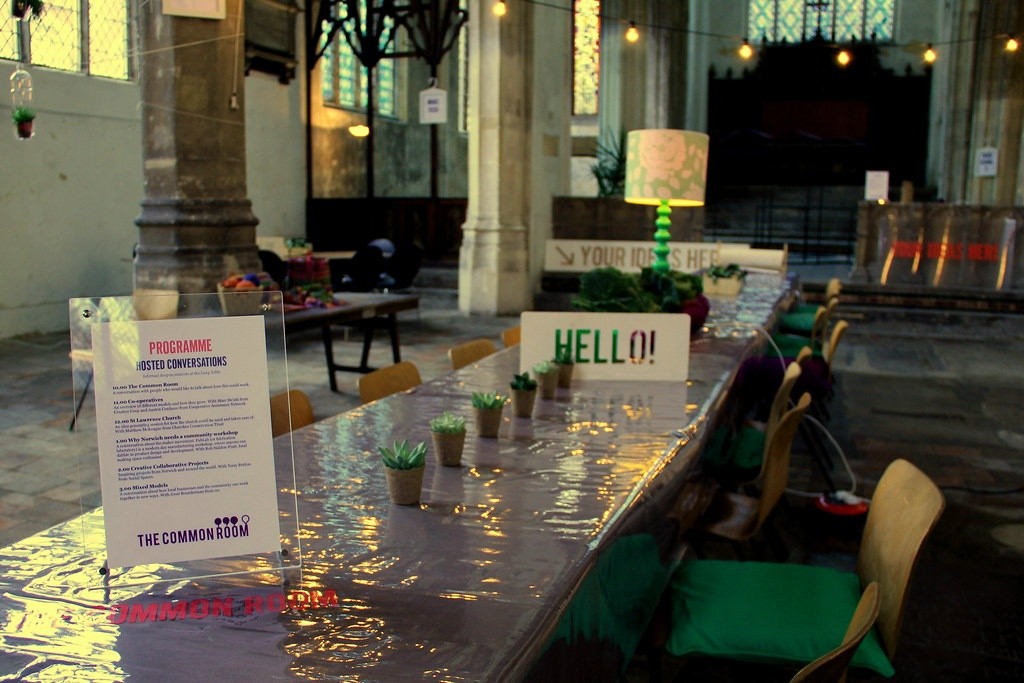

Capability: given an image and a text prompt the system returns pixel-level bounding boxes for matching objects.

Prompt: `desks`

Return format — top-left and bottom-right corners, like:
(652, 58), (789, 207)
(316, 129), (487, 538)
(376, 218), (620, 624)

(0, 263), (802, 683)
(254, 236), (358, 274)
(68, 291), (420, 433)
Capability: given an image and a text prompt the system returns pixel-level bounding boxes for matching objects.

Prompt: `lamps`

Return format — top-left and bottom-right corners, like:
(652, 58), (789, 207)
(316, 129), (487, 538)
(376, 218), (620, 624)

(624, 129), (711, 339)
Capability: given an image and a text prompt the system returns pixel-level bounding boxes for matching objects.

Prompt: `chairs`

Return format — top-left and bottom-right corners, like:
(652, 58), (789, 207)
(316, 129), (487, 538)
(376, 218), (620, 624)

(270, 325), (520, 438)
(692, 274), (851, 545)
(652, 456), (949, 683)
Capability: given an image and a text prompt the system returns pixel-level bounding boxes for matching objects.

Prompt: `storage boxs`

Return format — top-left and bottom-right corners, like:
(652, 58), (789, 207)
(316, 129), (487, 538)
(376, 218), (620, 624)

(216, 282), (263, 315)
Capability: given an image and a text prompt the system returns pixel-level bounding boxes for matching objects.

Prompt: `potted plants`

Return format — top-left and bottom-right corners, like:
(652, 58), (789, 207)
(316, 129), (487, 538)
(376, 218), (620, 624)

(12, 104), (36, 138)
(378, 354), (574, 505)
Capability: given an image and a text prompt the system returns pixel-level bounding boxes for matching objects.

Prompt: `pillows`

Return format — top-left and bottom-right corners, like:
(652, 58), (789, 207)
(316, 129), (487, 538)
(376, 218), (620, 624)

(664, 559), (896, 680)
(733, 426), (765, 467)
